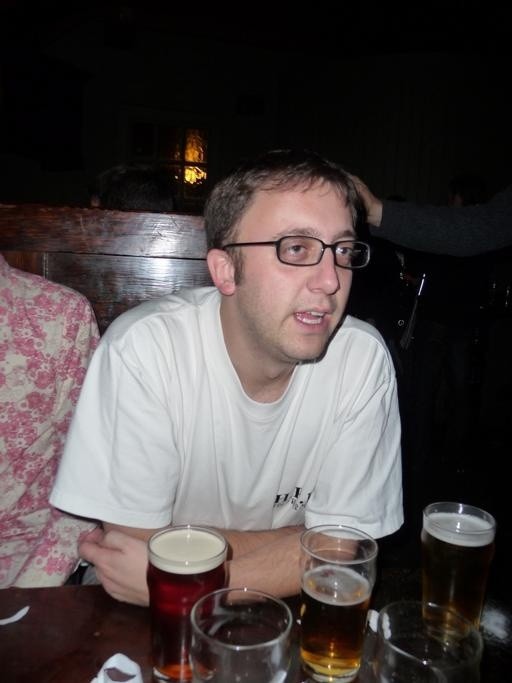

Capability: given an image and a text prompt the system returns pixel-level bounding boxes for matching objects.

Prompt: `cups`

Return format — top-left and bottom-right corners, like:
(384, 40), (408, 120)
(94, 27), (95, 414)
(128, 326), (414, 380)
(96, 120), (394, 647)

(373, 598), (481, 682)
(300, 524), (378, 682)
(148, 524), (229, 682)
(187, 589), (294, 682)
(419, 500), (494, 648)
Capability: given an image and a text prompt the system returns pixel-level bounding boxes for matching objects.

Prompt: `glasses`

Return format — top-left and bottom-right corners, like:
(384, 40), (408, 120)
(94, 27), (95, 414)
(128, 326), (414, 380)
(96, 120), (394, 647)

(223, 235), (372, 269)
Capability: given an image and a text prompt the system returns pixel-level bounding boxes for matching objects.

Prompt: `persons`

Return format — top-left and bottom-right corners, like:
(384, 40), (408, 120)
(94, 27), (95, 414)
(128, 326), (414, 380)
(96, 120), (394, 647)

(1, 253), (106, 592)
(319, 157), (512, 262)
(46, 149), (405, 609)
